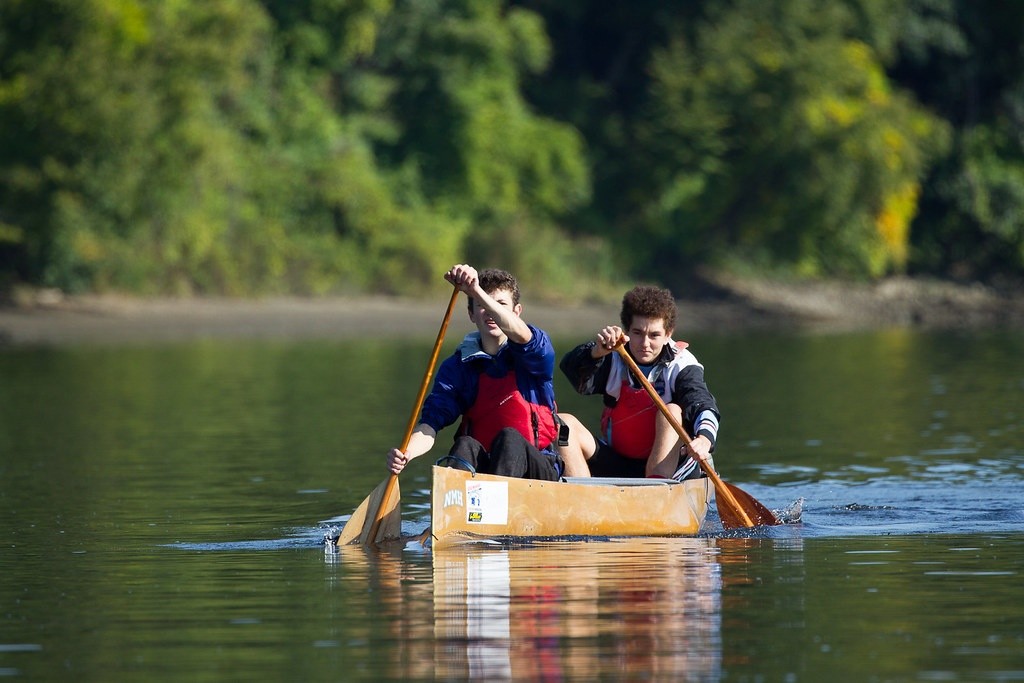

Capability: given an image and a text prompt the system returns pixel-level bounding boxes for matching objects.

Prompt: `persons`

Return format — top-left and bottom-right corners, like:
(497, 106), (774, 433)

(552, 285), (720, 482)
(385, 264), (565, 482)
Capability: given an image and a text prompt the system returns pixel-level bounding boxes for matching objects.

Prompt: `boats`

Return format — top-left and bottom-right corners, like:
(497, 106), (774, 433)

(419, 454), (721, 546)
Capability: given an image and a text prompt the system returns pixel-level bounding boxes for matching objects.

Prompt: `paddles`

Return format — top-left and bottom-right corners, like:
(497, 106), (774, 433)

(604, 334), (777, 530)
(335, 279), (463, 550)
